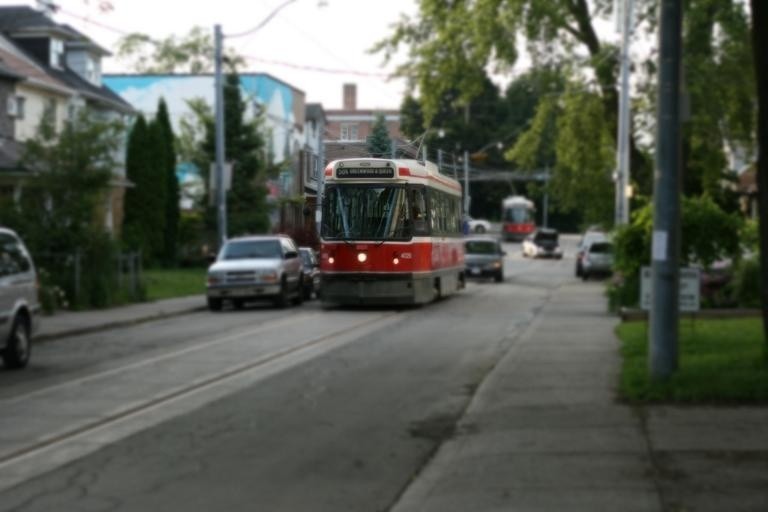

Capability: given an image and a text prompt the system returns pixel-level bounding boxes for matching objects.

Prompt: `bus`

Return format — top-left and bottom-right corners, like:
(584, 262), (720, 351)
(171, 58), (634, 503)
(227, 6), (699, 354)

(501, 193), (538, 241)
(315, 156), (467, 306)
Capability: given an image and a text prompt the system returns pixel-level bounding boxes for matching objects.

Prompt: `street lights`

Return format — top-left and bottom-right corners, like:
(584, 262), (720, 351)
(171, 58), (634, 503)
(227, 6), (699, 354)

(215, 0), (330, 251)
(464, 141), (504, 218)
(390, 128), (446, 159)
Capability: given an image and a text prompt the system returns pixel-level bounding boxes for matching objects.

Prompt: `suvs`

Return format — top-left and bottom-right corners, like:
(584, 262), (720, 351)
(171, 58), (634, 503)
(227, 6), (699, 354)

(206, 235), (304, 313)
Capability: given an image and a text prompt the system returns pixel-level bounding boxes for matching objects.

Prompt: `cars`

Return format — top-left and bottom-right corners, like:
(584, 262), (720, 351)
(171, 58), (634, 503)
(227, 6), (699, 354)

(578, 231), (602, 253)
(297, 246), (321, 302)
(466, 215), (491, 234)
(700, 253), (764, 294)
(0, 226), (44, 369)
(465, 236), (508, 283)
(522, 228), (564, 259)
(575, 243), (614, 281)
